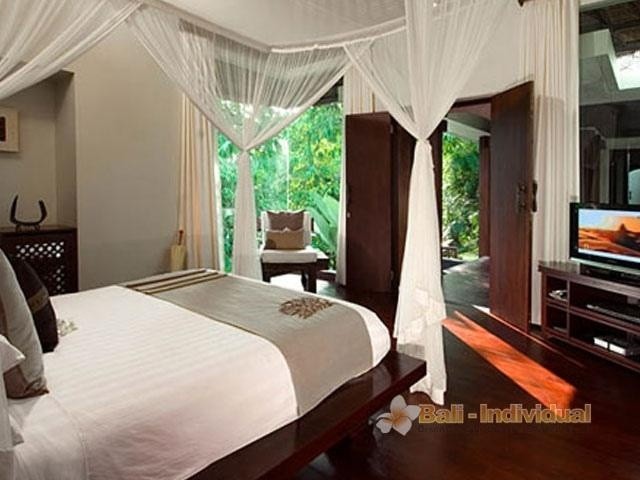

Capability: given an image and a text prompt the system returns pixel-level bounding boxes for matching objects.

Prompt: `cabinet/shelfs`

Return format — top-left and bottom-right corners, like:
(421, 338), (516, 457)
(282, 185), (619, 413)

(541, 273), (640, 374)
(1, 233), (78, 296)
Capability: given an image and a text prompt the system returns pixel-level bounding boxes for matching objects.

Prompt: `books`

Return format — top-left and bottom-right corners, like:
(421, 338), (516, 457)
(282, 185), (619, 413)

(593, 335), (614, 350)
(609, 337), (640, 357)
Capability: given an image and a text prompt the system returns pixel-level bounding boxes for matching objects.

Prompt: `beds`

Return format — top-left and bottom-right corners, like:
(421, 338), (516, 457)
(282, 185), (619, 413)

(0, 268), (428, 478)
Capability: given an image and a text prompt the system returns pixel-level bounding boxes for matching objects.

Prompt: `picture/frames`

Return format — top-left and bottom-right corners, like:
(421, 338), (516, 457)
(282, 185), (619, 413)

(0, 104), (22, 154)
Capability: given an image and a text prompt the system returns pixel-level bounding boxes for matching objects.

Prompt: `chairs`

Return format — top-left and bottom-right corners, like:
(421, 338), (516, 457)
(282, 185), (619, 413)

(257, 211), (318, 294)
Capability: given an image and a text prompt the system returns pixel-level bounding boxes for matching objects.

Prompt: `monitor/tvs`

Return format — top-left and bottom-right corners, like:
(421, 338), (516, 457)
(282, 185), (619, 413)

(568, 201), (640, 275)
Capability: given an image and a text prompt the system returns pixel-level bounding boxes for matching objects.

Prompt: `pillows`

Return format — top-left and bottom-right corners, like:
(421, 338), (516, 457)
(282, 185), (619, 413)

(261, 209), (308, 251)
(1, 248), (60, 400)
